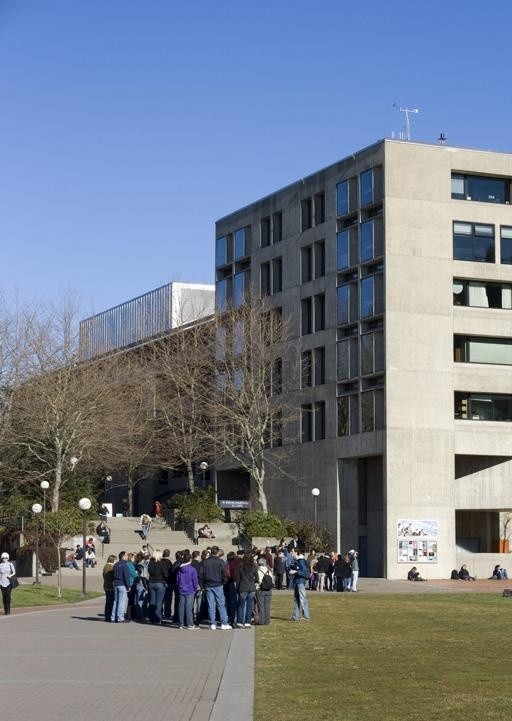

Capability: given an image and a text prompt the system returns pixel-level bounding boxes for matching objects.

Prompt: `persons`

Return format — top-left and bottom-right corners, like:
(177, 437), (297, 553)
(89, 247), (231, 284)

(120, 499), (130, 517)
(65, 538), (97, 570)
(140, 512), (152, 540)
(198, 524), (216, 539)
(102, 537), (359, 631)
(408, 567), (427, 581)
(97, 522), (111, 544)
(493, 565), (509, 580)
(459, 564), (476, 581)
(154, 501), (162, 518)
(0, 552), (16, 616)
(398, 522), (428, 537)
(98, 503), (109, 523)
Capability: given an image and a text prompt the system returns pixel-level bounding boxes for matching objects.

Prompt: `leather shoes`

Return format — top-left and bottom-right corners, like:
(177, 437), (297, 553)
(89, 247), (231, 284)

(117, 618), (131, 623)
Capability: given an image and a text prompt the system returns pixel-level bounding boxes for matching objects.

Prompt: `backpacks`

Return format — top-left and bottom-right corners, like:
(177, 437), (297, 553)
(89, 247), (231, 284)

(258, 569), (273, 591)
(298, 557), (310, 580)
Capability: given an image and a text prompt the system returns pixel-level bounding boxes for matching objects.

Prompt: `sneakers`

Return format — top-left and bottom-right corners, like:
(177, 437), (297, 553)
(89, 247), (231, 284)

(220, 623), (233, 630)
(187, 624), (201, 630)
(244, 623), (252, 628)
(179, 625), (184, 630)
(236, 623), (244, 628)
(209, 624), (216, 631)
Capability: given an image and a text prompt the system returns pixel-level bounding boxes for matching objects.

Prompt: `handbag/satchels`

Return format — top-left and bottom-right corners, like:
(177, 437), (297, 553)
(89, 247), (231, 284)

(9, 575), (19, 588)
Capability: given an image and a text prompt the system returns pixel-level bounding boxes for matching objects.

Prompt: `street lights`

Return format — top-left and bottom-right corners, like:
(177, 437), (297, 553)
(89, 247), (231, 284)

(311, 487), (321, 532)
(31, 503), (43, 588)
(78, 498), (91, 597)
(200, 461), (209, 489)
(41, 482), (49, 537)
(106, 476), (113, 503)
